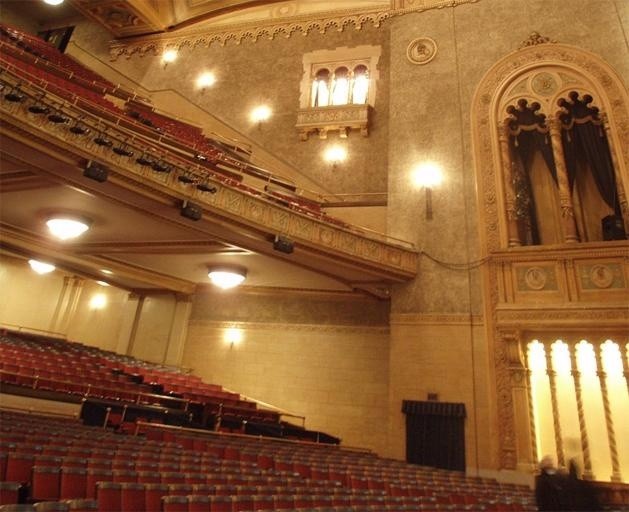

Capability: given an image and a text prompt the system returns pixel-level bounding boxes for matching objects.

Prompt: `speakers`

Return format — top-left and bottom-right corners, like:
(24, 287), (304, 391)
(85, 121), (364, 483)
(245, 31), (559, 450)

(83, 159), (109, 182)
(272, 234), (294, 252)
(180, 200), (201, 220)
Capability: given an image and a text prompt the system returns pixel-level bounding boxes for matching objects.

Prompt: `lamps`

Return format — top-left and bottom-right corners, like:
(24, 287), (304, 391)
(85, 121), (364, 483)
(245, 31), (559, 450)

(27, 257), (53, 275)
(45, 210), (94, 241)
(207, 266), (248, 294)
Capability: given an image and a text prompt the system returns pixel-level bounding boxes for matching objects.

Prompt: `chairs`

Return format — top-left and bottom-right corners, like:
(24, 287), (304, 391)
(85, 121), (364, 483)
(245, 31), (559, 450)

(227, 180), (359, 238)
